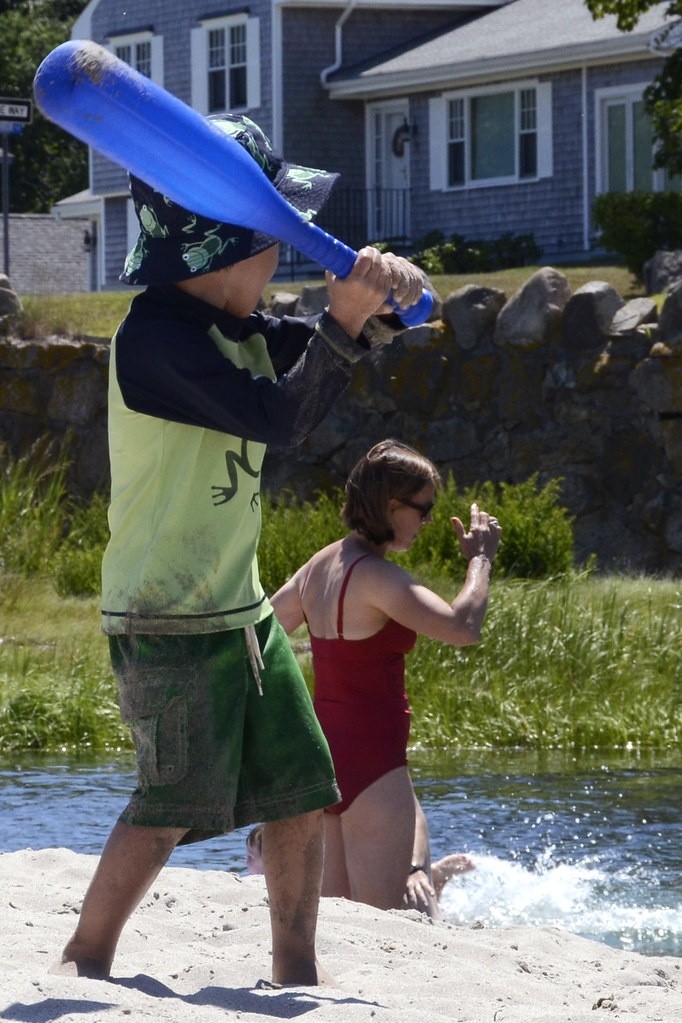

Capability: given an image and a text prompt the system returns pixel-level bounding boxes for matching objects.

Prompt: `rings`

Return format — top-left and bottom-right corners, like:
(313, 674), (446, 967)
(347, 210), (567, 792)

(489, 521), (498, 525)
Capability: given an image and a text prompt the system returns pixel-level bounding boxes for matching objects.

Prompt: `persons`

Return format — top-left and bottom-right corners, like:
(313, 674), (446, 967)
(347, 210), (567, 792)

(271, 441), (502, 911)
(56, 113), (426, 987)
(247, 783), (472, 922)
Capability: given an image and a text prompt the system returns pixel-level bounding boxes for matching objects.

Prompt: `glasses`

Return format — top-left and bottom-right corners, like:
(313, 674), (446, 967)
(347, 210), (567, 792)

(396, 496), (434, 520)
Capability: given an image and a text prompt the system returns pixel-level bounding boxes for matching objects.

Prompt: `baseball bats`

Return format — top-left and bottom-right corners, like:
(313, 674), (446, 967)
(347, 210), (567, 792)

(31, 38), (435, 328)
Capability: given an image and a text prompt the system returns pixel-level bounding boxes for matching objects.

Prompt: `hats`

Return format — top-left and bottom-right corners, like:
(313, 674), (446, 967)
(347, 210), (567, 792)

(117, 112), (342, 287)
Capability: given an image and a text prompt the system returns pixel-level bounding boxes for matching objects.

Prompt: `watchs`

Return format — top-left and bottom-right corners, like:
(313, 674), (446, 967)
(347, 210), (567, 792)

(410, 863), (427, 874)
